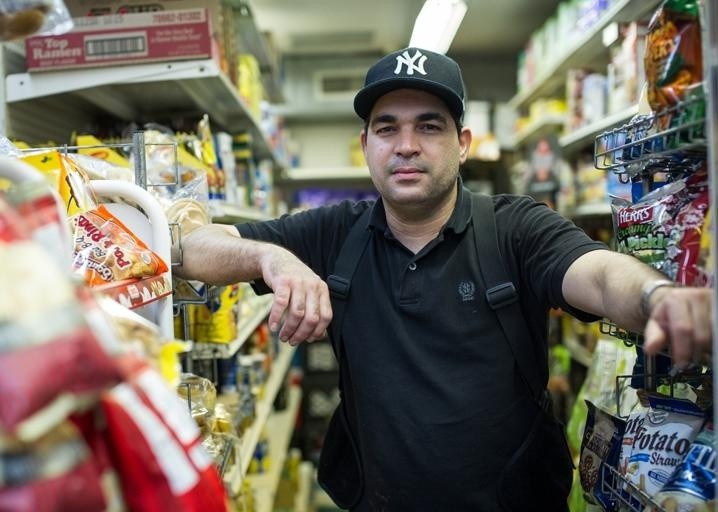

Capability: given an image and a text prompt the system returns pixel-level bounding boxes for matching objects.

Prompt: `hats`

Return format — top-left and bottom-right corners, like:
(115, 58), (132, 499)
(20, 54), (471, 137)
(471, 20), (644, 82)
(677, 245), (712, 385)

(354, 47), (465, 127)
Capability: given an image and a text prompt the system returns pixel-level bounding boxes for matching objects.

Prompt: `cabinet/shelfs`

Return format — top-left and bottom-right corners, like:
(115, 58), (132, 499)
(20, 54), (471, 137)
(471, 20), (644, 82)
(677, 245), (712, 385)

(0, 2), (718, 512)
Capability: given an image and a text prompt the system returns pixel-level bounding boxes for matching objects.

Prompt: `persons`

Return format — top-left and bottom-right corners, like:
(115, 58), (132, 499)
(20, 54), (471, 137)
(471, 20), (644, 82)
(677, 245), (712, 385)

(171, 46), (715, 512)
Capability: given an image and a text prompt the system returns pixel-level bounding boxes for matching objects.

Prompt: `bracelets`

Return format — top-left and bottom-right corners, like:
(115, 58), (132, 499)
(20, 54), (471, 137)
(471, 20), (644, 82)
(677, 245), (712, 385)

(642, 280), (674, 320)
